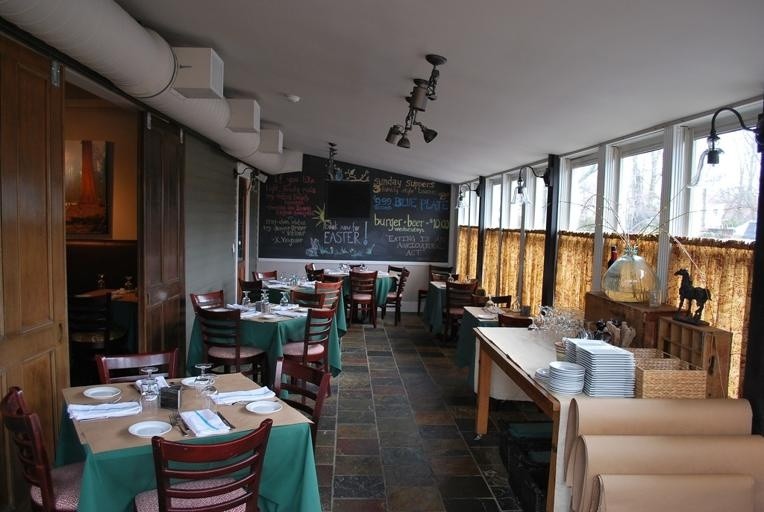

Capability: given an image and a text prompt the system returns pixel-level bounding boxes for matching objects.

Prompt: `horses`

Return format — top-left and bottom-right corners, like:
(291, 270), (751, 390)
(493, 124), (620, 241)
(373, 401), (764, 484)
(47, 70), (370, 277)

(674, 268), (712, 319)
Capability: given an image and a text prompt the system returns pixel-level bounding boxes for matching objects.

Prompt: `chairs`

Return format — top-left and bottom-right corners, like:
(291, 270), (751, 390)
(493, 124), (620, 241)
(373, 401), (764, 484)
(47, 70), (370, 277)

(266, 356), (331, 451)
(415, 261), (537, 391)
(279, 306), (340, 402)
(237, 245), (409, 327)
(189, 288), (229, 312)
(68, 291), (131, 383)
(194, 303), (267, 387)
(129, 418), (279, 512)
(0, 382), (87, 512)
(96, 349), (184, 384)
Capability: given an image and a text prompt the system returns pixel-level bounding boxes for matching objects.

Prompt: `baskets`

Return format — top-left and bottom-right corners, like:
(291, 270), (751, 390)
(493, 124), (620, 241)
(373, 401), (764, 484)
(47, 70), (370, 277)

(622, 347), (708, 398)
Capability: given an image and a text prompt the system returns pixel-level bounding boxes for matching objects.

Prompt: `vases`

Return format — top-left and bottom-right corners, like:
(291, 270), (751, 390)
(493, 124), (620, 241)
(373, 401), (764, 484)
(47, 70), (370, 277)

(597, 246), (656, 302)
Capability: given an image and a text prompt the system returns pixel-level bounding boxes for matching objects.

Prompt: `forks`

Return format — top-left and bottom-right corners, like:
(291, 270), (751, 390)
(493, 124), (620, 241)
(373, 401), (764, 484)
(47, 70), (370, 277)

(173, 411), (189, 430)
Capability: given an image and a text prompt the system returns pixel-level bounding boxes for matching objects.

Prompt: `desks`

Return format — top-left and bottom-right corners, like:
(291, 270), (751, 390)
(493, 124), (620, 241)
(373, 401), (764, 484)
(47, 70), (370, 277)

(471, 321), (685, 512)
(73, 285), (141, 314)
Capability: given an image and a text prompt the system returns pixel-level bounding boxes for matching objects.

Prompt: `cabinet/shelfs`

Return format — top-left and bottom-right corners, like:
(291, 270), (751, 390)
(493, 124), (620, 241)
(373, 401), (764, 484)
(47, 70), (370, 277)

(582, 289), (685, 349)
(656, 315), (733, 399)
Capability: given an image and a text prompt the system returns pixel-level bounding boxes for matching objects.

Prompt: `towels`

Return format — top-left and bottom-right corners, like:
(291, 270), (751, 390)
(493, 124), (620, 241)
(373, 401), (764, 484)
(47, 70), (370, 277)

(67, 402), (141, 422)
(179, 407), (230, 439)
(207, 384), (277, 405)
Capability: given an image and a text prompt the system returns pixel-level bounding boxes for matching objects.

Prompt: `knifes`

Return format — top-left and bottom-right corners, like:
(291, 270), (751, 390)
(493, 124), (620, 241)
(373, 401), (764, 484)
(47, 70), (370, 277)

(216, 410), (235, 430)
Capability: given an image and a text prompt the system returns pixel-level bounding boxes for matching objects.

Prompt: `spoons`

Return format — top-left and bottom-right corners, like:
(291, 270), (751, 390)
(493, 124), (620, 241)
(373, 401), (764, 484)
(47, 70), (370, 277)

(168, 416), (187, 437)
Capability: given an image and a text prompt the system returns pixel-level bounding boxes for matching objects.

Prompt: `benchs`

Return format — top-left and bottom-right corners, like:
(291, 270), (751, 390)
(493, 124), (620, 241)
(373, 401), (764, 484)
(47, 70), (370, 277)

(65, 240), (138, 331)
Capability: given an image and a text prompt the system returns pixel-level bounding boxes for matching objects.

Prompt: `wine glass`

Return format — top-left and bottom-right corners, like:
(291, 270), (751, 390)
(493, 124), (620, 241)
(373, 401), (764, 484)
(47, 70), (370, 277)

(241, 290), (251, 309)
(194, 362), (211, 386)
(140, 367), (158, 399)
(95, 272), (106, 291)
(447, 272), (454, 283)
(124, 274), (133, 290)
(260, 288), (271, 314)
(278, 291), (288, 311)
(280, 272), (306, 286)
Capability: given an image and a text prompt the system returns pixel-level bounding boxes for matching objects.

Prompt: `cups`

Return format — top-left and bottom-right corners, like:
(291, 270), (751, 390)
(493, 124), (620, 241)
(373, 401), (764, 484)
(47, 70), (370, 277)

(528, 306), (592, 339)
(521, 305), (532, 318)
(646, 286), (663, 309)
(359, 263), (368, 272)
(512, 302), (520, 313)
(337, 263), (349, 275)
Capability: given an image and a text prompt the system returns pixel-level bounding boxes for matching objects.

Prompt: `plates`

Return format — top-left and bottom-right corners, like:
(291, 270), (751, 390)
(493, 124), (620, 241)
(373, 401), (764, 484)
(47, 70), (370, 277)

(81, 386), (121, 399)
(135, 377), (161, 390)
(127, 420), (171, 439)
(244, 400), (282, 415)
(534, 338), (637, 400)
(179, 376), (216, 387)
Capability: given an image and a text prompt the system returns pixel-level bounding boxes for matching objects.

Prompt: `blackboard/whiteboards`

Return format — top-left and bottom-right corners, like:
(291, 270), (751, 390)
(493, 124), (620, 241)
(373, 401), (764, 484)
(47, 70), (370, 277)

(256, 154), (456, 268)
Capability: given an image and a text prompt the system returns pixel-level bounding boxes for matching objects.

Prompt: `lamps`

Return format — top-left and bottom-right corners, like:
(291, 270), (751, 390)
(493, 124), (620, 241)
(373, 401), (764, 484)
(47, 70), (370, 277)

(510, 160), (554, 206)
(408, 52), (448, 112)
(234, 165), (259, 194)
(678, 101), (764, 194)
(450, 179), (481, 211)
(320, 142), (345, 183)
(383, 94), (440, 150)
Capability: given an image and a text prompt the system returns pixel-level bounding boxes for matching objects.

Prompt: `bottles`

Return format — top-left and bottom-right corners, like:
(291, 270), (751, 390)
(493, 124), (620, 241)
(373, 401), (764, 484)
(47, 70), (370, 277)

(605, 245), (618, 273)
(486, 294), (494, 313)
(255, 300), (260, 311)
